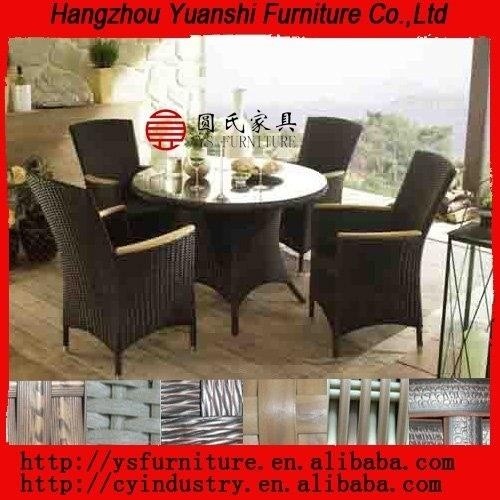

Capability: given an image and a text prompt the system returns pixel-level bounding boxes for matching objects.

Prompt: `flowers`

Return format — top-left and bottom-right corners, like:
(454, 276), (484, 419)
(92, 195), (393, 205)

(7, 165), (27, 228)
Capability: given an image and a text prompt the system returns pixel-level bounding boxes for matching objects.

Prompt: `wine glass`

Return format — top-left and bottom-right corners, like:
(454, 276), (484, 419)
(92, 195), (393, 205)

(187, 147), (208, 193)
(252, 144), (271, 191)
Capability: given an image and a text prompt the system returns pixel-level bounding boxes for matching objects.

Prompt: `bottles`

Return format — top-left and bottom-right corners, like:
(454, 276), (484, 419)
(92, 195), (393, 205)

(8, 64), (33, 114)
(225, 87), (253, 160)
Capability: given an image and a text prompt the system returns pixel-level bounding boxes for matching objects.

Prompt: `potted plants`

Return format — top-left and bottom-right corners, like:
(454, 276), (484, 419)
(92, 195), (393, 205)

(87, 38), (119, 104)
(20, 156), (60, 261)
(478, 189), (492, 229)
(184, 123), (213, 185)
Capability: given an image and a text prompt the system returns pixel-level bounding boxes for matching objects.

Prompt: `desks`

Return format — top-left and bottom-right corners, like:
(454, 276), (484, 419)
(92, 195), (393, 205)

(131, 157), (329, 336)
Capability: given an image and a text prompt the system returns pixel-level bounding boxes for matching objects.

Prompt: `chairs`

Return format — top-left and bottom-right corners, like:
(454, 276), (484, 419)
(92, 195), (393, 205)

(310, 150), (456, 357)
(279, 117), (364, 273)
(69, 119), (152, 210)
(25, 172), (197, 376)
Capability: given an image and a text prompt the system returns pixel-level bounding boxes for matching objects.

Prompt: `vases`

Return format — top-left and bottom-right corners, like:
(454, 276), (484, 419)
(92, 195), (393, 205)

(9, 230), (20, 268)
(220, 86), (253, 158)
